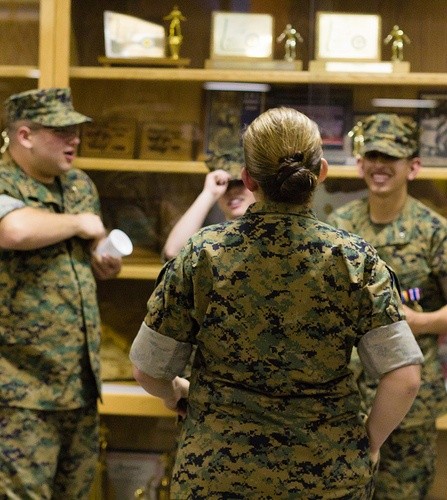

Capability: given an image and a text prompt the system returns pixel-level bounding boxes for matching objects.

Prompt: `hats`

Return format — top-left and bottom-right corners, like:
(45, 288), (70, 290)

(204, 148), (246, 182)
(4, 87), (94, 127)
(357, 114), (420, 161)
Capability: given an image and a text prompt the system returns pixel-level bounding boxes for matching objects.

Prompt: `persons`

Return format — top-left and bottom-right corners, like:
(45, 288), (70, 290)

(1, 88), (122, 499)
(129, 107), (425, 499)
(162, 137), (256, 382)
(277, 24), (303, 62)
(325, 114), (447, 499)
(384, 26), (413, 61)
(162, 6), (187, 36)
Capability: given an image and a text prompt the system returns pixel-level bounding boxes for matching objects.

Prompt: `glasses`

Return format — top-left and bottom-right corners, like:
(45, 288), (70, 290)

(25, 125), (78, 139)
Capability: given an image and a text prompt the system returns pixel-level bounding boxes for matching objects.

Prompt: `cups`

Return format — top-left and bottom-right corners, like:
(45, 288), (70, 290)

(95, 229), (133, 259)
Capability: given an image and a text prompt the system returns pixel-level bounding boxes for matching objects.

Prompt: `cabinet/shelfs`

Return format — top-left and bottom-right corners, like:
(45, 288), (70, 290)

(52, 0), (447, 451)
(0, 0), (53, 90)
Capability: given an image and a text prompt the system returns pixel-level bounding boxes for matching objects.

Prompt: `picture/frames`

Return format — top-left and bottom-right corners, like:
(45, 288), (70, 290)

(98, 195), (161, 259)
(264, 85), (356, 165)
(415, 90), (447, 169)
